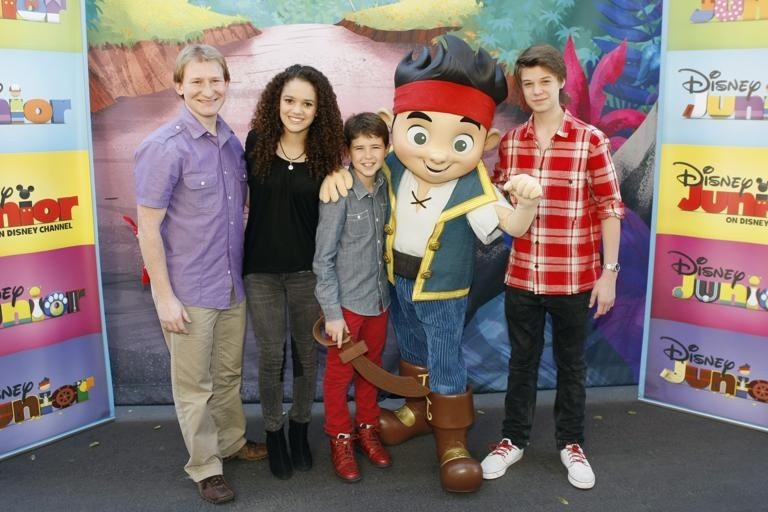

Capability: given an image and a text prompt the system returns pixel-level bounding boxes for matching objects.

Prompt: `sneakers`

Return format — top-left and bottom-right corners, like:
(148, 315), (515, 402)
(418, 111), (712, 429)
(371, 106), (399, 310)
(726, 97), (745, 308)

(480, 438), (524, 480)
(223, 439), (268, 461)
(330, 432), (360, 483)
(356, 423), (391, 467)
(560, 442), (596, 489)
(197, 474), (234, 504)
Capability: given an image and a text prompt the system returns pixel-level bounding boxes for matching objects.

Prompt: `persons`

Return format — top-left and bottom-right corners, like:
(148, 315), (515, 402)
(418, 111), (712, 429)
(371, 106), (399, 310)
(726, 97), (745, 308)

(480, 43), (628, 491)
(241, 61), (348, 482)
(311, 110), (395, 482)
(130, 41), (270, 506)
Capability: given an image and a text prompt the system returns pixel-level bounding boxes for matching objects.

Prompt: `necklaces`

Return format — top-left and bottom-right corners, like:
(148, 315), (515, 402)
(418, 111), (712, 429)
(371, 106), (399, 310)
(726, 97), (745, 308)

(275, 138), (308, 174)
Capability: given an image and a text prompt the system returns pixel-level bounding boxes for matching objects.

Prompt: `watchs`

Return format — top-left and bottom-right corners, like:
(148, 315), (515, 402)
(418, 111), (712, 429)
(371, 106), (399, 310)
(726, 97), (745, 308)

(600, 262), (622, 274)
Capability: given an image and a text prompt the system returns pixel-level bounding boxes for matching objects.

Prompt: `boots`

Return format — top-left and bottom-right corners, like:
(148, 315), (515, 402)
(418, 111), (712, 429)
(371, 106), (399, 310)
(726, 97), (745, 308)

(265, 422), (292, 480)
(289, 419), (312, 470)
